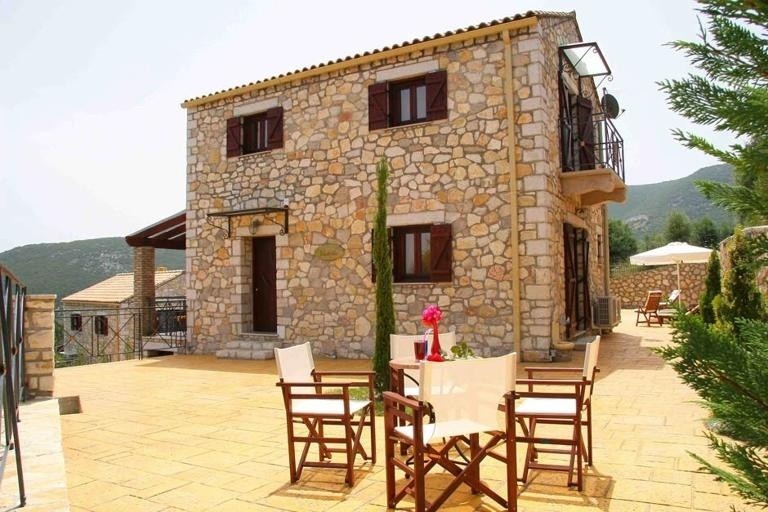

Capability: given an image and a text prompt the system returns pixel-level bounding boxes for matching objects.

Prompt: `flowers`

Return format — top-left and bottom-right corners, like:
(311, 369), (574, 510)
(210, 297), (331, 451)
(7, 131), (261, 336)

(422, 305), (441, 331)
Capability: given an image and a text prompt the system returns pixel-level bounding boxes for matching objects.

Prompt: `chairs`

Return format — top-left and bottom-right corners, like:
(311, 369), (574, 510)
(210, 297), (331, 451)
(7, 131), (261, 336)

(509, 335), (601, 492)
(388, 333), (457, 455)
(273, 341), (377, 487)
(634, 290), (699, 327)
(381, 352), (519, 512)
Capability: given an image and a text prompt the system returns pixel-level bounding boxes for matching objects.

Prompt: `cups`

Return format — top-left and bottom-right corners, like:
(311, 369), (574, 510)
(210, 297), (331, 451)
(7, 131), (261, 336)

(414, 339), (428, 361)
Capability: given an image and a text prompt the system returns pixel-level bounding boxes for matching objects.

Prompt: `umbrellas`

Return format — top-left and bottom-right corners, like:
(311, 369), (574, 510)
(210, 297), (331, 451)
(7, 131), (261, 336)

(629, 241), (721, 313)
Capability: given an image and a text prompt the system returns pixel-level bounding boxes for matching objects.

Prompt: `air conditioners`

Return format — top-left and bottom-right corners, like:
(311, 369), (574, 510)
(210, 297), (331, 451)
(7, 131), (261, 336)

(596, 295), (621, 329)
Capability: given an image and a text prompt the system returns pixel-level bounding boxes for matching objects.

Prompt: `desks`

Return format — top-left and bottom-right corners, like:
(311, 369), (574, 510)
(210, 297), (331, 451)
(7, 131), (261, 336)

(390, 356), (492, 479)
